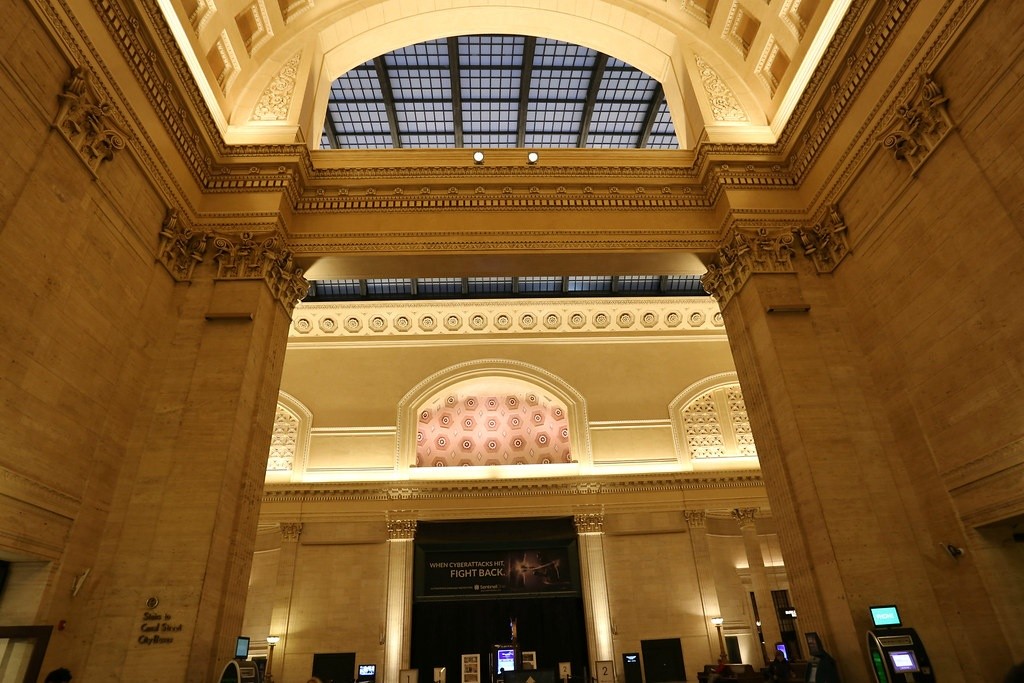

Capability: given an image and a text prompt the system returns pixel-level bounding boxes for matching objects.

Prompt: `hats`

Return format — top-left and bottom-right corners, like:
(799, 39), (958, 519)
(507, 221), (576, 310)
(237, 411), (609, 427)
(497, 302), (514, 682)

(776, 650), (784, 656)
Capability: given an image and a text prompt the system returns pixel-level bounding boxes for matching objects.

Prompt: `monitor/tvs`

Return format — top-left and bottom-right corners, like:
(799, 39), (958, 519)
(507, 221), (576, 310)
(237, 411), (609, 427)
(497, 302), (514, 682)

(869, 604), (902, 629)
(889, 651), (920, 673)
(235, 637), (250, 660)
(358, 665), (376, 676)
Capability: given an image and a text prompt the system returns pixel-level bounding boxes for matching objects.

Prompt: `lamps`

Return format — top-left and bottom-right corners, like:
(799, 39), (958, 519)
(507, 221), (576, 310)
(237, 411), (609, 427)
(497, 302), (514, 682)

(473, 151), (485, 164)
(528, 151), (539, 164)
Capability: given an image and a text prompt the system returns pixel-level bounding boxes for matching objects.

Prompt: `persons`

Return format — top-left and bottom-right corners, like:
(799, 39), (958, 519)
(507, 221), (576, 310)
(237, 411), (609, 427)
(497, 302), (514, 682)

(770, 650), (791, 683)
(44, 668), (72, 683)
(308, 677), (321, 683)
(708, 659), (724, 683)
(500, 668), (504, 673)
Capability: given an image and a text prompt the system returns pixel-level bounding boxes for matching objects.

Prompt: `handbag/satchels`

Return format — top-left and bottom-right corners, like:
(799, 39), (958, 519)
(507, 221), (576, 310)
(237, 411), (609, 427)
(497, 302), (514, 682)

(768, 665), (776, 683)
